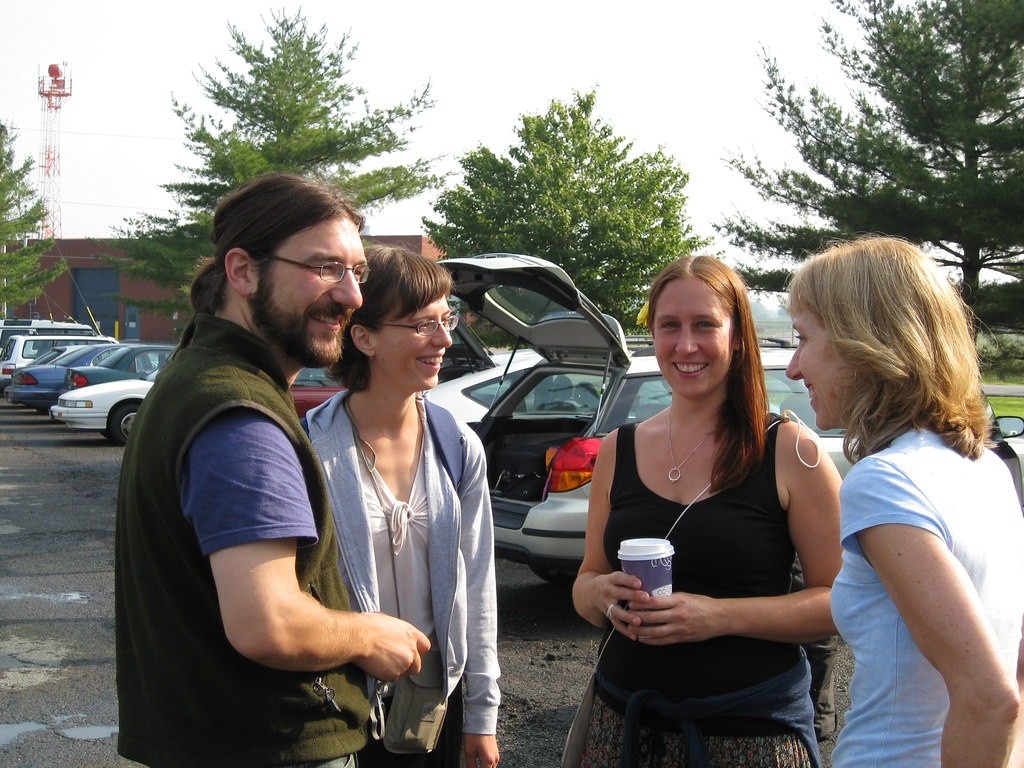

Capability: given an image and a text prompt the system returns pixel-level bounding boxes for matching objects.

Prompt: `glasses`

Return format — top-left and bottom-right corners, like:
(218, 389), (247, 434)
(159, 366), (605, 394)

(382, 315), (459, 336)
(267, 254), (372, 284)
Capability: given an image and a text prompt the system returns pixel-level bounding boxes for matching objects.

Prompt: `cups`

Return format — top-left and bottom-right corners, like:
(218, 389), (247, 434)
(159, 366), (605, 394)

(617, 538), (675, 626)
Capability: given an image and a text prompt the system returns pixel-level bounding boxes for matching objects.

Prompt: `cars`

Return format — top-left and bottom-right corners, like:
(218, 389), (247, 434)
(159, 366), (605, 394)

(26, 346), (85, 368)
(48, 368), (161, 446)
(57, 344), (177, 398)
(3, 343), (157, 408)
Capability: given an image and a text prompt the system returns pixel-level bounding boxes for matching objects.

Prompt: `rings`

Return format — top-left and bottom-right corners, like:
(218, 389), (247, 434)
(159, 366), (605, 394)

(607, 605), (613, 618)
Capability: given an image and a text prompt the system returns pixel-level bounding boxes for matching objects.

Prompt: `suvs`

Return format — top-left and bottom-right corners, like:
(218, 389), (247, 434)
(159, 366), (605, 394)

(288, 252), (1024, 590)
(0, 318), (97, 355)
(0, 335), (121, 390)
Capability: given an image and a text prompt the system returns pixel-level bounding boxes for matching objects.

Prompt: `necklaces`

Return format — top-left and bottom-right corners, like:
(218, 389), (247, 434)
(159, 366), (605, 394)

(667, 409), (710, 482)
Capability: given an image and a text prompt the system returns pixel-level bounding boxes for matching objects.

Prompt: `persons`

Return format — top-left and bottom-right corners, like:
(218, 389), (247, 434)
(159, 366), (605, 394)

(572, 255), (844, 768)
(115, 173), (432, 768)
(299, 244), (502, 768)
(785, 238), (1024, 768)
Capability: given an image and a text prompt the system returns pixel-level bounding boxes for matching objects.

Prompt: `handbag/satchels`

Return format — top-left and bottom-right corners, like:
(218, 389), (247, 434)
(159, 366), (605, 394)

(377, 651), (448, 757)
